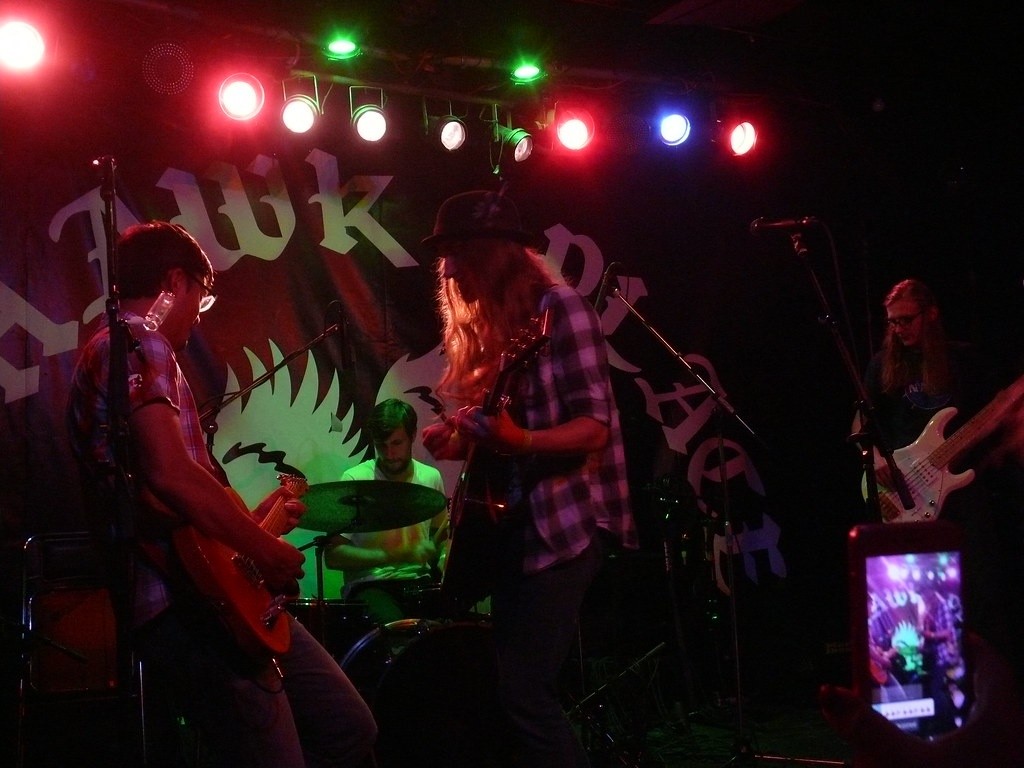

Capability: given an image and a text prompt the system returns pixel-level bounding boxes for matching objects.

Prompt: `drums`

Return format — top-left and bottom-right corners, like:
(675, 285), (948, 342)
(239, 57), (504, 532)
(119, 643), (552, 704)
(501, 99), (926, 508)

(288, 597), (366, 660)
(340, 619), (521, 767)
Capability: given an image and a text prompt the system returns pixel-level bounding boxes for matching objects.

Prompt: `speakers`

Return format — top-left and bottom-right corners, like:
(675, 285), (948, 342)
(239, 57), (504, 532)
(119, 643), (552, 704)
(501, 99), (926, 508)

(20, 530), (126, 710)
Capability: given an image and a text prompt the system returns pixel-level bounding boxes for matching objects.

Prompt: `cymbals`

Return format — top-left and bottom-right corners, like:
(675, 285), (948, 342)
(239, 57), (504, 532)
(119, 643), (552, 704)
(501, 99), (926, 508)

(296, 479), (449, 532)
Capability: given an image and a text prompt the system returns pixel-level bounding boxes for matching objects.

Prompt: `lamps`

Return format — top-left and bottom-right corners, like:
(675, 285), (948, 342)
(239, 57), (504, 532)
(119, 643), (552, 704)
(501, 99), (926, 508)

(415, 91), (466, 149)
(343, 84), (389, 146)
(273, 69), (323, 134)
(479, 105), (535, 169)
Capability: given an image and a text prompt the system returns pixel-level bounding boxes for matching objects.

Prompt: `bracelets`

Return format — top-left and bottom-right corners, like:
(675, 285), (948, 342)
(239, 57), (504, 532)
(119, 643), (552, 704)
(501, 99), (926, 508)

(509, 429), (532, 454)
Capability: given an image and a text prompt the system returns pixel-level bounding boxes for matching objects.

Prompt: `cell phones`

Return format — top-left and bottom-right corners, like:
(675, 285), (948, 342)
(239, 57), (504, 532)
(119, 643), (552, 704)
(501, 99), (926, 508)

(849, 520), (977, 740)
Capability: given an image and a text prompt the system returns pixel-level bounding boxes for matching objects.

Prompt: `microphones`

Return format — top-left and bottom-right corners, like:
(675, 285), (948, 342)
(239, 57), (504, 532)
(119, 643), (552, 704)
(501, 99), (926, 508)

(93, 155), (115, 176)
(338, 304), (350, 357)
(750, 216), (821, 238)
(594, 262), (616, 313)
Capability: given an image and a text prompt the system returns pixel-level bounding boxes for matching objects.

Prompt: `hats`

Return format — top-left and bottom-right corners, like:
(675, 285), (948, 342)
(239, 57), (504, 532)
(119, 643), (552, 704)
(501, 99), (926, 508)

(420, 191), (533, 247)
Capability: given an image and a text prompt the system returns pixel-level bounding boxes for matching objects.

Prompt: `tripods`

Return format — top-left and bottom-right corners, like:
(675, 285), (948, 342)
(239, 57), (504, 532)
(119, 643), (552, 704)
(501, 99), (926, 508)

(608, 280), (847, 768)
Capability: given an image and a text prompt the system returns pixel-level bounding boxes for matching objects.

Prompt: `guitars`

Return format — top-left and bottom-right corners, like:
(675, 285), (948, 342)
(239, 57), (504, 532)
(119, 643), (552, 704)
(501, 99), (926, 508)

(439, 307), (552, 598)
(173, 470), (307, 655)
(863, 378), (1024, 521)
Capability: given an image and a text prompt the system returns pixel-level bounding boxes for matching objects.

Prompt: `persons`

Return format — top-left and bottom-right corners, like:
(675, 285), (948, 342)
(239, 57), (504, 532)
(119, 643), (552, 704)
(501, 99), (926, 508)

(868, 576), (961, 699)
(64, 224), (378, 767)
(326, 399), (453, 628)
(851, 280), (1024, 485)
(422, 189), (638, 768)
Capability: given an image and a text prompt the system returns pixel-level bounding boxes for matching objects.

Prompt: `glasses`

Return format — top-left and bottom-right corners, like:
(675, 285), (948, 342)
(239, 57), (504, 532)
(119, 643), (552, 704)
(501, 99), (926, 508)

(190, 272), (217, 312)
(886, 307), (922, 331)
